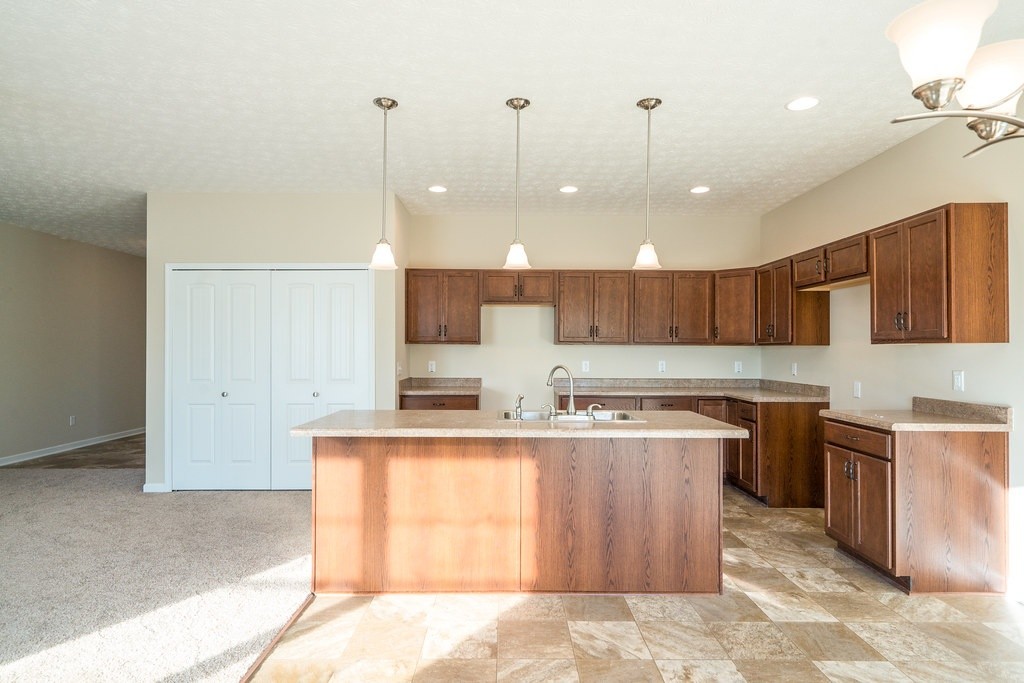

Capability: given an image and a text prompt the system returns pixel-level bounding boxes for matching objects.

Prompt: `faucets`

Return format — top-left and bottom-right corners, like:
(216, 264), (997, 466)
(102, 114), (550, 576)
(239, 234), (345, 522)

(546, 364), (577, 421)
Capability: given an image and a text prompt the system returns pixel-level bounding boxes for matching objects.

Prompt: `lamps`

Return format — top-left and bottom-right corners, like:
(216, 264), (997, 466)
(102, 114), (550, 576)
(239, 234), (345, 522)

(888, 0), (1024, 159)
(367, 97), (400, 271)
(632, 98), (663, 271)
(501, 98), (531, 270)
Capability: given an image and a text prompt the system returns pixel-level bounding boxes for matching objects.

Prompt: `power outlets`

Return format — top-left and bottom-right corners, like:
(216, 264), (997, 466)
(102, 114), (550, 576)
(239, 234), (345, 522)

(953, 370), (964, 392)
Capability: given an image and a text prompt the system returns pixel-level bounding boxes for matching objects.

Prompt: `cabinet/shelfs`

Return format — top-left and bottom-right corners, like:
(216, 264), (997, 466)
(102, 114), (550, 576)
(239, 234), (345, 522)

(825, 418), (1010, 596)
(405, 203), (1009, 347)
(400, 395), (830, 509)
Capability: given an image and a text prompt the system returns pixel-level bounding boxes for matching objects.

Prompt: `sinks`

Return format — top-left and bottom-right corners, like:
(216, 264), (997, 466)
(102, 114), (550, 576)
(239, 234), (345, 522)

(562, 409), (647, 423)
(496, 408), (570, 422)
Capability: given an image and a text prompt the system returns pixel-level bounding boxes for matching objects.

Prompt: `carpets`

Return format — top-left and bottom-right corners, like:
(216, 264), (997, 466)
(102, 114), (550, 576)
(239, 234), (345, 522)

(0, 469), (314, 683)
